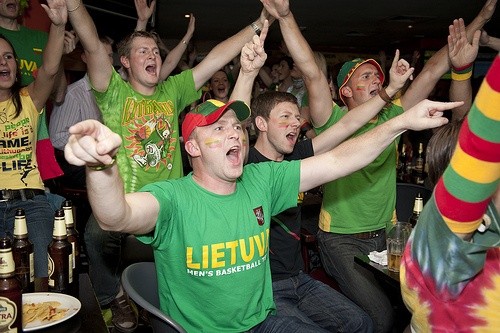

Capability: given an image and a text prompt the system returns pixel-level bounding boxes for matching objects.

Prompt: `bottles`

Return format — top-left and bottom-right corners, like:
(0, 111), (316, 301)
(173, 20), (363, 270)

(47, 209), (73, 295)
(396, 141), (425, 183)
(11, 208), (35, 293)
(408, 193), (424, 229)
(0, 237), (23, 333)
(61, 199), (80, 296)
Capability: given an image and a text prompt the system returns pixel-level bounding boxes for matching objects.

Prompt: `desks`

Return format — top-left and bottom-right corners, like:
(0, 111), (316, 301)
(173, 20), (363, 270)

(0, 270), (110, 333)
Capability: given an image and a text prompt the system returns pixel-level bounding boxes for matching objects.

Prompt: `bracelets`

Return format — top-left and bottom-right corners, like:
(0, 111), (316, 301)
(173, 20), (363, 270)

(67, 0), (82, 13)
(251, 22), (261, 36)
(379, 88), (392, 104)
(181, 40), (188, 45)
(451, 63), (473, 80)
(85, 154), (117, 171)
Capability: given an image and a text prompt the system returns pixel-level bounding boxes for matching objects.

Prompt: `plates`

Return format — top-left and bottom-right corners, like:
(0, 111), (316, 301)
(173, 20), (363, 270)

(22, 292), (81, 331)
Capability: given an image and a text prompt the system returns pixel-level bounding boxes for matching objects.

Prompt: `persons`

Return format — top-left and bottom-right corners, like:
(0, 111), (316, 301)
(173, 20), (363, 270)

(0, 0), (500, 333)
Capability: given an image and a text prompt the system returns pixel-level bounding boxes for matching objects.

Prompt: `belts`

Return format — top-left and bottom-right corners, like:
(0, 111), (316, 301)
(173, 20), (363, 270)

(0, 188), (45, 202)
(353, 230), (381, 239)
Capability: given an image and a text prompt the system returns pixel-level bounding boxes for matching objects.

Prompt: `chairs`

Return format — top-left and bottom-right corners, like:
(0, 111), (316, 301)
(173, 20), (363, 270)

(121, 182), (432, 333)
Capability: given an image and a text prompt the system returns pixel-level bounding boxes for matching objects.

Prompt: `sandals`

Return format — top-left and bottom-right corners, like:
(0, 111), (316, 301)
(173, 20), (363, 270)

(108, 285), (139, 332)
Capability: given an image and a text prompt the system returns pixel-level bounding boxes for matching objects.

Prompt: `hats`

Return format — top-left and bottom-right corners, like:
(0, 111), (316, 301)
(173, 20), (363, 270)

(336, 57), (385, 107)
(182, 99), (252, 144)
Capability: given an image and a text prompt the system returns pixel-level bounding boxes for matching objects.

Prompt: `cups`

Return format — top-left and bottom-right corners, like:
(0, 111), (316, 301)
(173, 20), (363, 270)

(385, 221), (412, 273)
